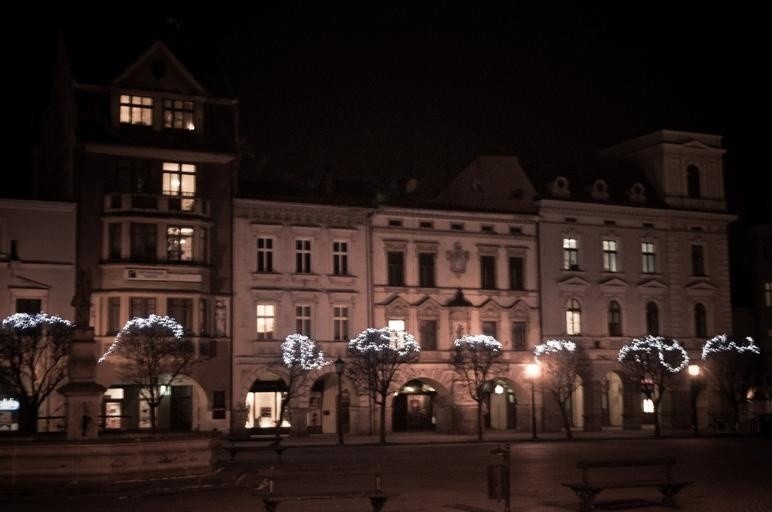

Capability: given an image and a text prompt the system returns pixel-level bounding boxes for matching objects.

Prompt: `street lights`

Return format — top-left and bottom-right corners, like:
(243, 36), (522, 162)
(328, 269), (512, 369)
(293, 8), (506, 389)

(525, 358), (542, 440)
(687, 356), (702, 435)
(334, 353), (348, 443)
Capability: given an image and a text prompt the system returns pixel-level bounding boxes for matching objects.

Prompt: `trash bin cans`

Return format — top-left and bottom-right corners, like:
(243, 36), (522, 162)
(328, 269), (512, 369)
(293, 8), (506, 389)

(488, 442), (510, 504)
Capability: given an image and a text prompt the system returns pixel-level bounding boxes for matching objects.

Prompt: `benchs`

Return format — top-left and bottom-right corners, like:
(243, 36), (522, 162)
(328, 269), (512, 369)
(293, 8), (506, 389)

(564, 461), (682, 511)
